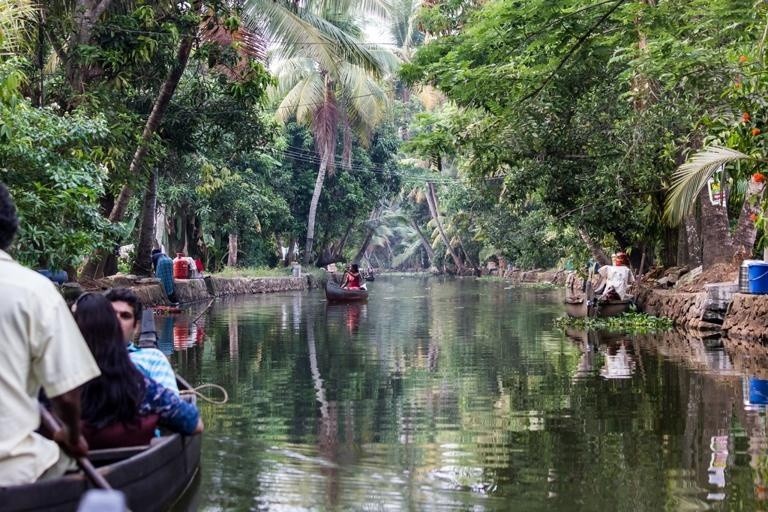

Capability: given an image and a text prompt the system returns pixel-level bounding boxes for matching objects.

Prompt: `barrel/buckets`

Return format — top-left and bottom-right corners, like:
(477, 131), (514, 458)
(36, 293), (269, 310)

(748, 375), (767, 403)
(738, 258), (762, 292)
(292, 265), (302, 277)
(289, 261), (298, 267)
(742, 377), (766, 416)
(748, 262), (767, 294)
(610, 252), (617, 267)
(566, 256), (574, 268)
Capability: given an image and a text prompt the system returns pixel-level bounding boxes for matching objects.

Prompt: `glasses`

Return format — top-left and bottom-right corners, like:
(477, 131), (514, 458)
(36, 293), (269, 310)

(116, 310), (132, 320)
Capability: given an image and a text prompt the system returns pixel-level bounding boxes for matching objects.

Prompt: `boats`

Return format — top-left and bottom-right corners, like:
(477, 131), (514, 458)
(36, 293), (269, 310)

(1, 309), (200, 512)
(563, 295), (634, 319)
(325, 274), (374, 299)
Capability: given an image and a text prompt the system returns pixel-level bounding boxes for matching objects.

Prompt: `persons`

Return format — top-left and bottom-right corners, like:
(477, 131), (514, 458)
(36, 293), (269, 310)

(95, 285), (181, 437)
(615, 251), (627, 266)
(150, 248), (180, 304)
(345, 303), (360, 342)
(157, 313), (176, 358)
(31, 290), (206, 463)
(1, 180), (104, 489)
(339, 263), (363, 291)
